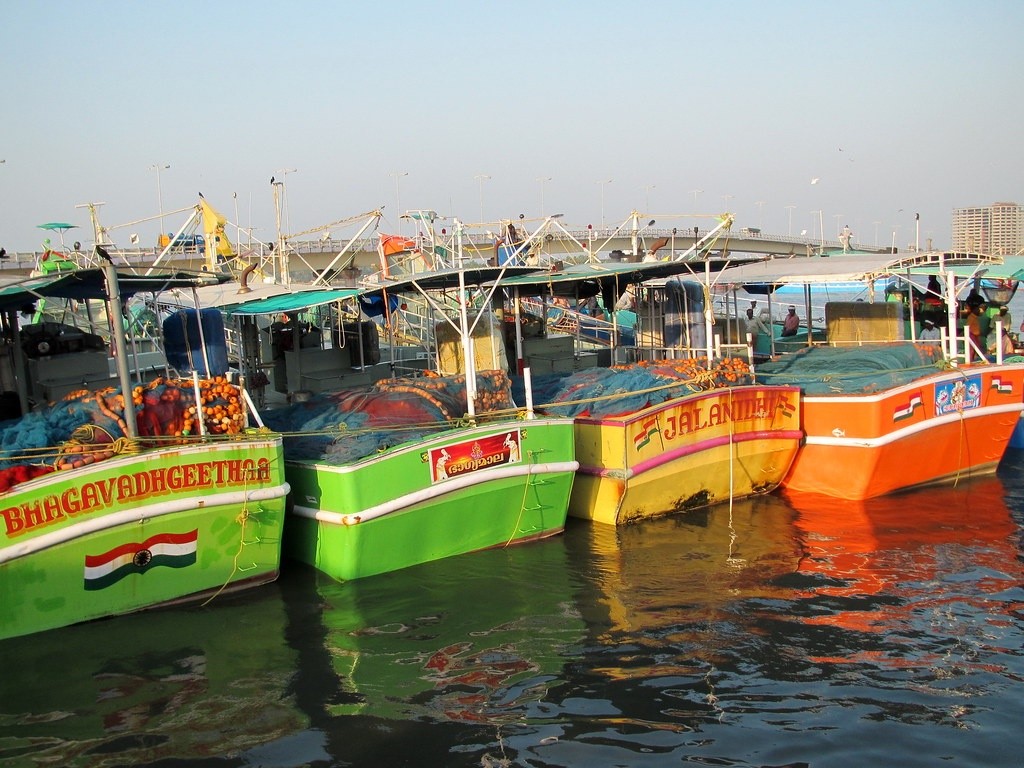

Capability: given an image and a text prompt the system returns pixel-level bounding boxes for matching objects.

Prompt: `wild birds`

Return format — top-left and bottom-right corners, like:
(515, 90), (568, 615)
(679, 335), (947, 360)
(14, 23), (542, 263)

(270, 176), (275, 184)
(801, 230), (807, 236)
(810, 177), (820, 185)
(96, 245), (113, 265)
(199, 192), (204, 199)
(0, 247), (6, 258)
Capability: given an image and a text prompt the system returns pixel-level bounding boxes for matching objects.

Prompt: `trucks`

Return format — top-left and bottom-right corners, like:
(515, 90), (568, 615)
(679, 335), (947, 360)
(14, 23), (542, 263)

(159, 234), (206, 252)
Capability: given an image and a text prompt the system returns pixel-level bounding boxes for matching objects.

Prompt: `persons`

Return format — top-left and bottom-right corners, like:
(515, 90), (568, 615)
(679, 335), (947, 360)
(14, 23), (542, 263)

(744, 308), (771, 340)
(965, 289), (984, 307)
(966, 305), (982, 362)
(918, 319), (940, 346)
(987, 328), (1014, 355)
(781, 305), (799, 337)
(746, 300), (762, 319)
(986, 305), (1011, 334)
(924, 275), (941, 299)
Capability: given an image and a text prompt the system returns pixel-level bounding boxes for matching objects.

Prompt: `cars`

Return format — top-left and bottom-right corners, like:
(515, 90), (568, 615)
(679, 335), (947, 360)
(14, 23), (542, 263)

(21, 322), (104, 356)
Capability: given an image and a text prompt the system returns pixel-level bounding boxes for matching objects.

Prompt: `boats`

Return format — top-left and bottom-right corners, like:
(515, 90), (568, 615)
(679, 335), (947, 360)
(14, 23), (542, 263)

(0, 179), (1023, 640)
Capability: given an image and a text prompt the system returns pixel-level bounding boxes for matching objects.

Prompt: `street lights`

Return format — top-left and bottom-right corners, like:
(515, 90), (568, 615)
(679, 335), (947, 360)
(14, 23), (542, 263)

(873, 221), (881, 246)
(719, 194), (735, 212)
(150, 163), (170, 234)
(595, 180), (613, 229)
(642, 185), (656, 224)
(274, 168), (298, 235)
(831, 215), (845, 238)
(688, 190), (705, 215)
(474, 175), (492, 231)
(388, 173), (409, 235)
(782, 205), (796, 238)
(536, 178), (552, 218)
